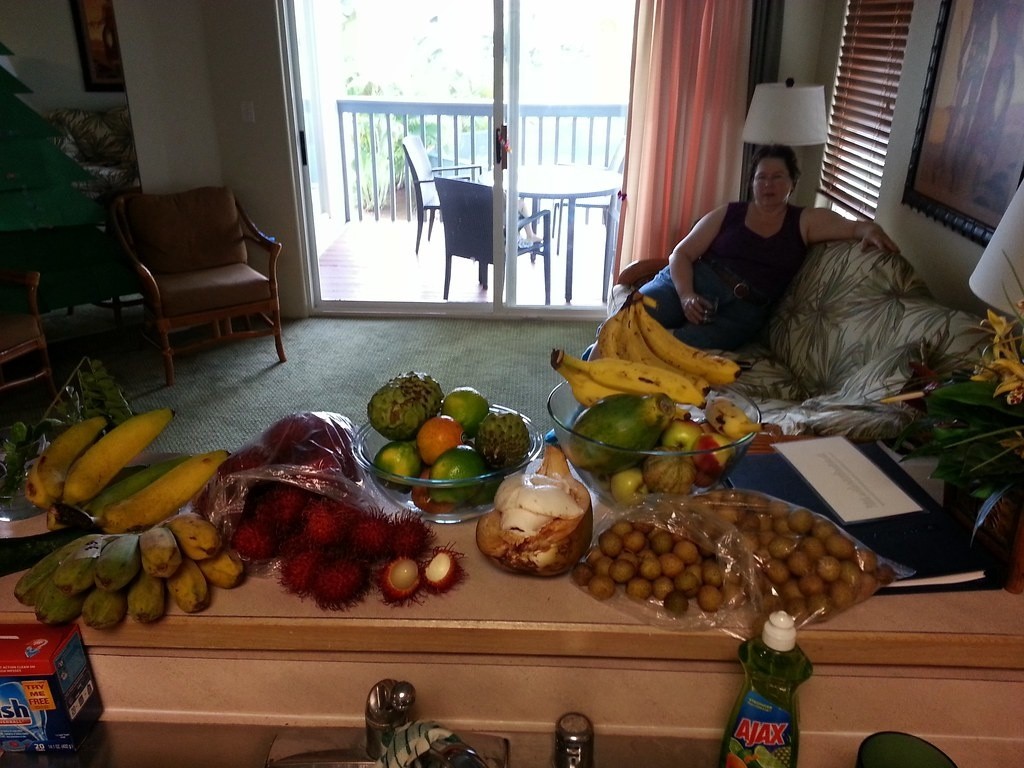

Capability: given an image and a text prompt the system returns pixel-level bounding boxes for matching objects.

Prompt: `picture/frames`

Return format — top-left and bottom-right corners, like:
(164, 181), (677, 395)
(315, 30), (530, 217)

(900, 0), (1023, 247)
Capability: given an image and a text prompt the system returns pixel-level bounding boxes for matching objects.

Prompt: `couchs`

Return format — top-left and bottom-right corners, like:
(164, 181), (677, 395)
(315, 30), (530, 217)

(613, 235), (998, 453)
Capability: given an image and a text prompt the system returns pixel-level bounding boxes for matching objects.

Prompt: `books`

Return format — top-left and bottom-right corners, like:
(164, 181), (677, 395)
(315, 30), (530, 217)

(721, 442), (1004, 595)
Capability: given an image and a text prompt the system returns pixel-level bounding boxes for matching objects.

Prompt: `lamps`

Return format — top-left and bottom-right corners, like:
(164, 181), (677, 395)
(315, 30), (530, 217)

(740, 78), (831, 152)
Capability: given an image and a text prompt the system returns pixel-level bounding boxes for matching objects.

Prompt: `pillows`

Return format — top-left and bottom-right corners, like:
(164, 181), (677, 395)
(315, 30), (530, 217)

(131, 187), (249, 274)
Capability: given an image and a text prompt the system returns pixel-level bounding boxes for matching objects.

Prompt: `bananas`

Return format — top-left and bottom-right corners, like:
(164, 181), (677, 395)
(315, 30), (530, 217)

(550, 289), (763, 438)
(14, 408), (242, 630)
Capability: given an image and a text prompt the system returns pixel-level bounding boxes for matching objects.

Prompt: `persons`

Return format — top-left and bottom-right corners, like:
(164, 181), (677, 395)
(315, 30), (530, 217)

(503, 191), (543, 248)
(545, 144), (901, 443)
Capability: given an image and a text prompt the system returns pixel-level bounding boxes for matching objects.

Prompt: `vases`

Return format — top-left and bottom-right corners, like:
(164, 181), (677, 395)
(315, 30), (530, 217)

(941, 469), (1024, 559)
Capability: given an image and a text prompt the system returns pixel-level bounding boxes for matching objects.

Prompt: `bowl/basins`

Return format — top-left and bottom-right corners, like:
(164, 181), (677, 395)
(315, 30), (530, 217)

(351, 403), (543, 523)
(547, 378), (762, 505)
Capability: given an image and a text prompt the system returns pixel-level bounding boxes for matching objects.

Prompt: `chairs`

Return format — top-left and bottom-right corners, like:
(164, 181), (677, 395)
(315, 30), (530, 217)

(108, 192), (288, 387)
(0, 268), (64, 406)
(404, 134), (624, 298)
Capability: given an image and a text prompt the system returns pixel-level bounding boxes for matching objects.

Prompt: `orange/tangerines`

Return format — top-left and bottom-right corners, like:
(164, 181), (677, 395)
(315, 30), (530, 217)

(372, 387), (489, 515)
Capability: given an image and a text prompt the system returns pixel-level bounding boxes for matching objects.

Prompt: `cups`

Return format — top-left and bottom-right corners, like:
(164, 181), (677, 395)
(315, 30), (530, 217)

(854, 731), (958, 768)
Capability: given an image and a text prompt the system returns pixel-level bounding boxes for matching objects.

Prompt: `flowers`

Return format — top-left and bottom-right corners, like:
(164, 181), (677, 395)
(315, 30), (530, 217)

(888, 300), (1024, 552)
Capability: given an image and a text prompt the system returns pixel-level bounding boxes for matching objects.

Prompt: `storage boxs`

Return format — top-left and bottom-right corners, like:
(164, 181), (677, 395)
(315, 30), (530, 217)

(1, 622), (105, 754)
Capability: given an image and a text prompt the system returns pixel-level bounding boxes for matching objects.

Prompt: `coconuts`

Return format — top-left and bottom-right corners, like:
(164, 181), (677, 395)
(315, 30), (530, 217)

(476, 446), (593, 576)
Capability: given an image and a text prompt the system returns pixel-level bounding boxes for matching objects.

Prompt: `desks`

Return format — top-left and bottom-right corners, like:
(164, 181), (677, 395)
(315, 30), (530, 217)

(478, 166), (621, 304)
(0, 486), (1023, 768)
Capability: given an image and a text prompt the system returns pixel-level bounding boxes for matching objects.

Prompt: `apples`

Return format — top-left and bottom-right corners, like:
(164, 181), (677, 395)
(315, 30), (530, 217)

(594, 419), (735, 502)
